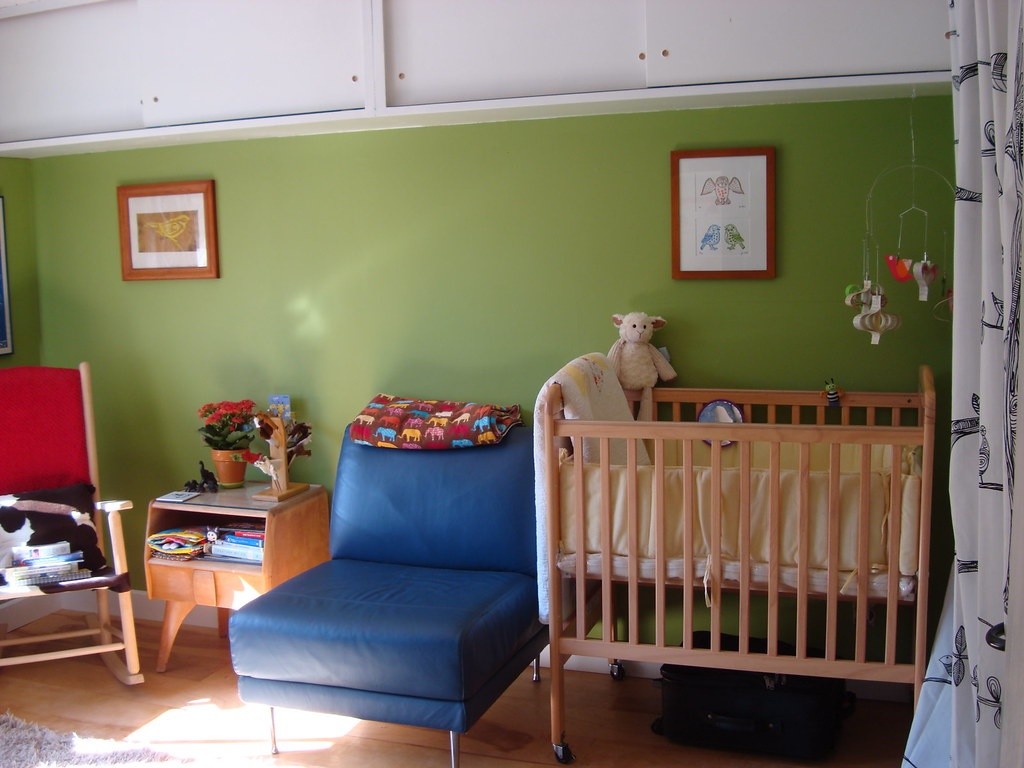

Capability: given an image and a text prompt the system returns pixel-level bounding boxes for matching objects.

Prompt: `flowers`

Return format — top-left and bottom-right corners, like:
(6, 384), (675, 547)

(196, 399), (261, 463)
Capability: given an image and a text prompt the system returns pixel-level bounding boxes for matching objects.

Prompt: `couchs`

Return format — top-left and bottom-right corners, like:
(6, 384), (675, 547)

(229, 422), (550, 768)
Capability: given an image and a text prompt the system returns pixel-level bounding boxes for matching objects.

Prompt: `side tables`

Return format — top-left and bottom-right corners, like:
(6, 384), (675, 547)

(143, 480), (331, 672)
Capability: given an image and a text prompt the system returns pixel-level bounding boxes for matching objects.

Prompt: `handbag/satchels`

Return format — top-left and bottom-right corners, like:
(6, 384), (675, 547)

(650, 631), (855, 764)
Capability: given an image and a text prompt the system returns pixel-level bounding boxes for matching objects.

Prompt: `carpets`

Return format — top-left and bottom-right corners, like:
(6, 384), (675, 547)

(0, 711), (198, 768)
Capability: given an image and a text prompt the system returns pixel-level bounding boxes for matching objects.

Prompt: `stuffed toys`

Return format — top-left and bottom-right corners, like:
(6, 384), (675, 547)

(820, 378), (844, 405)
(608, 312), (676, 421)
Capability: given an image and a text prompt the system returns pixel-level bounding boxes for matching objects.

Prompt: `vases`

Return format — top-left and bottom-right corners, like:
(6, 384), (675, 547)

(210, 449), (248, 488)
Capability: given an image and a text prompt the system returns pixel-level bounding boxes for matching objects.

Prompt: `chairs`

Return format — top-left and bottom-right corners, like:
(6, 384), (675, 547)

(0, 361), (144, 685)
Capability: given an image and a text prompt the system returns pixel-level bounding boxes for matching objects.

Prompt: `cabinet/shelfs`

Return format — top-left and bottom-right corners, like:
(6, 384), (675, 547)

(0, 0), (367, 145)
(382, 0), (952, 108)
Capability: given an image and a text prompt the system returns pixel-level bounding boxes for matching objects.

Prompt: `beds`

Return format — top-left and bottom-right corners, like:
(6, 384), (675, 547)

(544, 352), (936, 764)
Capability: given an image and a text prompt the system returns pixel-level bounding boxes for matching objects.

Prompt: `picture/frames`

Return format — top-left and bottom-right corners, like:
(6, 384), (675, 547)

(116, 178), (219, 280)
(0, 196), (14, 356)
(670, 146), (776, 279)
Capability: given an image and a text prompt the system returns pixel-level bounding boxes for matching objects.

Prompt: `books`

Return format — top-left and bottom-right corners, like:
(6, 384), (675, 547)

(0, 541), (92, 586)
(204, 522), (265, 566)
(156, 491), (200, 503)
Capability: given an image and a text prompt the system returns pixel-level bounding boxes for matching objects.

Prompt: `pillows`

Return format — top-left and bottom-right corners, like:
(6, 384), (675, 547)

(0, 483), (112, 587)
